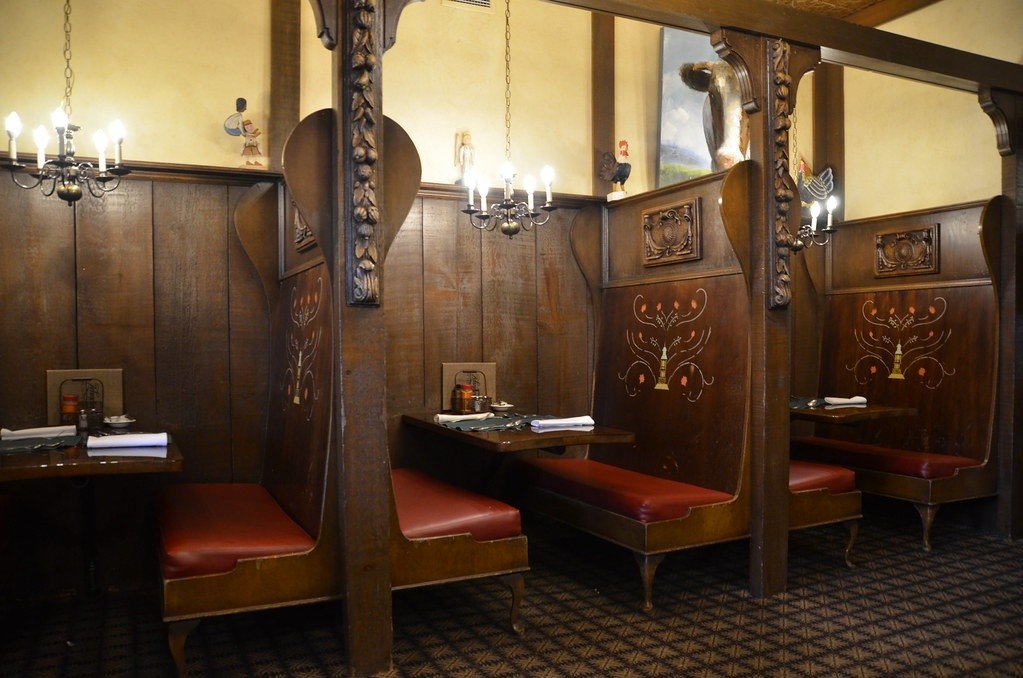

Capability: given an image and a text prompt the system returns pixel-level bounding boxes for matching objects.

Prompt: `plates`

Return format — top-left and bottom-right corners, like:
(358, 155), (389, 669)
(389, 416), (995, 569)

(490, 403), (514, 411)
(103, 415), (136, 427)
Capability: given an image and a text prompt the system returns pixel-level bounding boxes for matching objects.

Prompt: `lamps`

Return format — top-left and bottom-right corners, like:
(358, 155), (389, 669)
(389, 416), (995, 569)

(0, 0), (130, 204)
(791, 108), (838, 255)
(454, 0), (557, 239)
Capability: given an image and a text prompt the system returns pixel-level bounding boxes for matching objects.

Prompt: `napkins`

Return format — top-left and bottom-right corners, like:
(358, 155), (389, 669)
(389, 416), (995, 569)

(1, 426), (79, 441)
(86, 431), (169, 447)
(433, 411), (495, 424)
(529, 415), (596, 432)
(824, 395), (867, 406)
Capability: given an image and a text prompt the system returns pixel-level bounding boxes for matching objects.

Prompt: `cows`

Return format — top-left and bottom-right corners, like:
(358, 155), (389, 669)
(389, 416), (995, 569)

(679, 60), (749, 172)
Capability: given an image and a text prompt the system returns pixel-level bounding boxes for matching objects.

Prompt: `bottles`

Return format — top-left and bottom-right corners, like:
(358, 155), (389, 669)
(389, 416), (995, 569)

(79, 409), (87, 437)
(62, 393), (80, 423)
(454, 384), (461, 412)
(90, 409), (98, 434)
(460, 384), (473, 413)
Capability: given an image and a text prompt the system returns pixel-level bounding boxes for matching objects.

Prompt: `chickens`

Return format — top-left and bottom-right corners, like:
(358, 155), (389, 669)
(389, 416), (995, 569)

(799, 156), (835, 209)
(599, 140), (632, 192)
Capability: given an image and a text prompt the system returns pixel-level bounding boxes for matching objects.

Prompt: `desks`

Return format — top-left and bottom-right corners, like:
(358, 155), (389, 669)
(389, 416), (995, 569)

(399, 411), (637, 452)
(789, 398), (919, 423)
(0, 427), (183, 605)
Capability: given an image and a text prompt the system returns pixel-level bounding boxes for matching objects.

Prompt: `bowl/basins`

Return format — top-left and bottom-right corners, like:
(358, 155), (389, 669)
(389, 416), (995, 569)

(471, 395), (492, 412)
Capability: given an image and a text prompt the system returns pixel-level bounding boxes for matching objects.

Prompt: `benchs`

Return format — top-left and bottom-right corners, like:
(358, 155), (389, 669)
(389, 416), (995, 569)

(783, 459), (861, 569)
(791, 434), (996, 554)
(525, 457), (750, 613)
(388, 467), (528, 636)
(153, 482), (346, 678)
